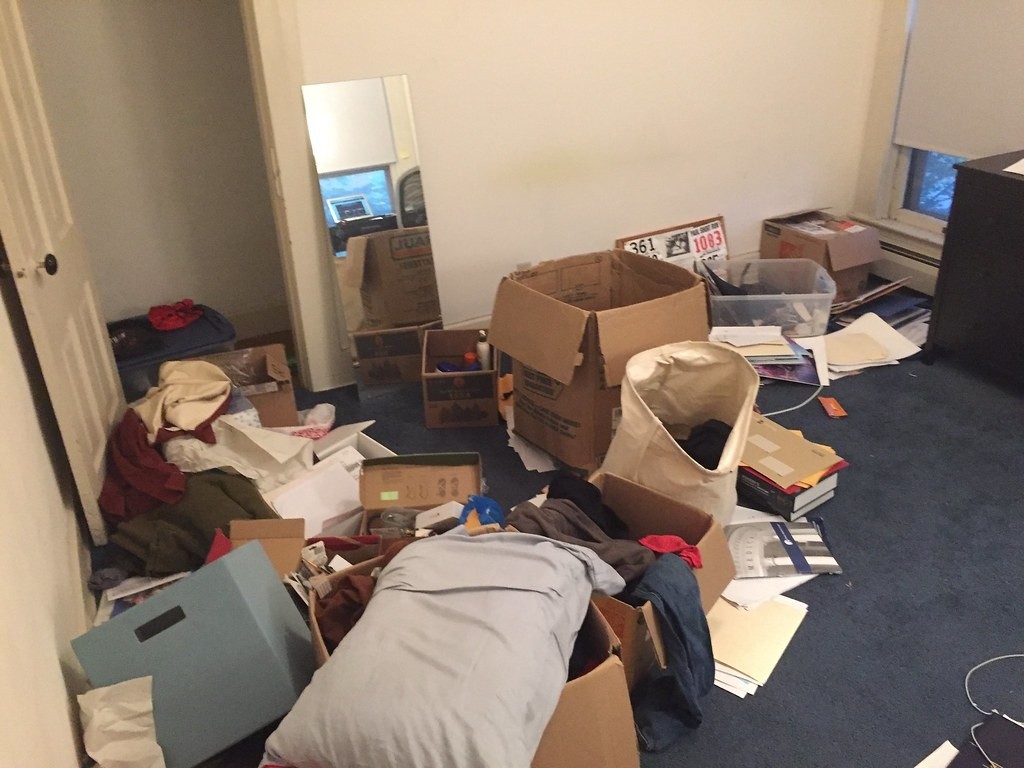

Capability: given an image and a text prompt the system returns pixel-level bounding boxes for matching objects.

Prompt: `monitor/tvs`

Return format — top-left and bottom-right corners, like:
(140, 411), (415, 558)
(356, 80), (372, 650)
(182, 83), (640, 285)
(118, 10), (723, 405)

(317, 163), (395, 228)
(325, 195), (373, 223)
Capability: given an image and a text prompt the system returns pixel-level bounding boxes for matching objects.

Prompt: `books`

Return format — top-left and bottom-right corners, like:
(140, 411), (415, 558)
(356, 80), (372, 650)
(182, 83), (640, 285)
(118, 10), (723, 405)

(708, 276), (931, 386)
(736, 410), (849, 522)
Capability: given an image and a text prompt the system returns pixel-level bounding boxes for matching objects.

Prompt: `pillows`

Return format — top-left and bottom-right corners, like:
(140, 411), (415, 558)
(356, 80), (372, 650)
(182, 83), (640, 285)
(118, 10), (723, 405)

(258, 525), (626, 768)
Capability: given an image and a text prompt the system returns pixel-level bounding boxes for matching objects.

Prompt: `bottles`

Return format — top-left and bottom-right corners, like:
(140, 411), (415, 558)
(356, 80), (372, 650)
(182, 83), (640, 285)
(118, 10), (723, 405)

(477, 330), (491, 372)
(463, 352), (480, 371)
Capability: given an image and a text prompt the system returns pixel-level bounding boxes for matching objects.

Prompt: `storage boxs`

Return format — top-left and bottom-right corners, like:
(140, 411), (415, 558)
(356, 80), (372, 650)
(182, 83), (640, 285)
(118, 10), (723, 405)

(486, 249), (714, 469)
(694, 257), (836, 340)
(759, 207), (888, 305)
(70, 455), (737, 768)
(106, 302), (237, 406)
(183, 344), (298, 428)
(338, 223), (444, 388)
(421, 328), (500, 428)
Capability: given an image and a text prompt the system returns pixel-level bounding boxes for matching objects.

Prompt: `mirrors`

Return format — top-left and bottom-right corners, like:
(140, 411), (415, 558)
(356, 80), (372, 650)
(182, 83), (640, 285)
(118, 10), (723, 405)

(301, 74), (444, 402)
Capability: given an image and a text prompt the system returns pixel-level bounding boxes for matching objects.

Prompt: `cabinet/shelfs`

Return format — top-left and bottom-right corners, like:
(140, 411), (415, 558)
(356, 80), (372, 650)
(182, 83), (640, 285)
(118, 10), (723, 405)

(926, 150), (1023, 394)
